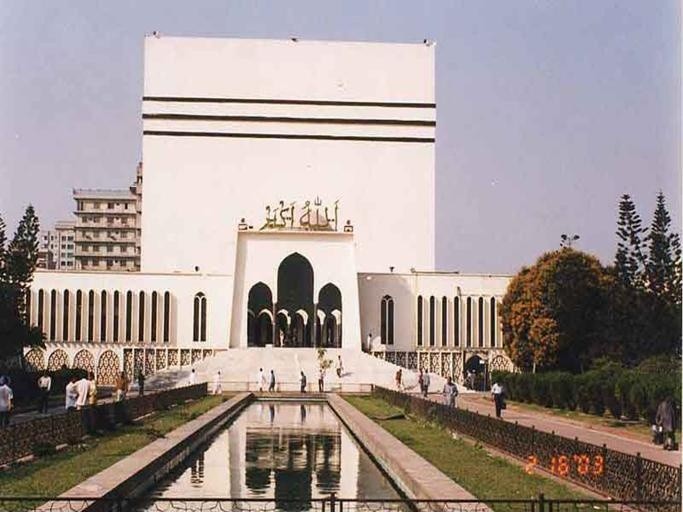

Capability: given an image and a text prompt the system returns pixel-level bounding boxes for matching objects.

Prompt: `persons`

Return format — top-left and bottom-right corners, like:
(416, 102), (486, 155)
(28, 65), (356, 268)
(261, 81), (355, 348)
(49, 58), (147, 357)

(490, 378), (505, 419)
(396, 369), (403, 388)
(316, 344), (347, 393)
(421, 369), (430, 396)
(299, 371), (307, 392)
(258, 368), (267, 391)
(649, 417), (665, 446)
(0, 364), (148, 428)
(654, 393), (678, 450)
(213, 370), (222, 394)
(444, 376), (458, 408)
(187, 369), (196, 385)
(268, 370), (276, 392)
(418, 367), (425, 392)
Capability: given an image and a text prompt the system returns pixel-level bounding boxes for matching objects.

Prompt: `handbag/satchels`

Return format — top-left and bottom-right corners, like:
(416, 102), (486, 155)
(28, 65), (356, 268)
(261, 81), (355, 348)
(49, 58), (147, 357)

(653, 424), (663, 445)
(501, 401), (506, 409)
